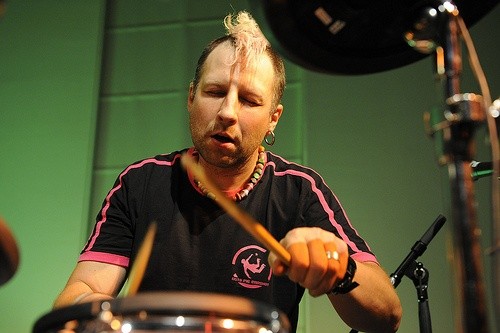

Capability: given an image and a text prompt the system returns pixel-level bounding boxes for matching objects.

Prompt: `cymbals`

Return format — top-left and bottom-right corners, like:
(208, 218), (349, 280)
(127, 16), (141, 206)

(255, 0), (500, 76)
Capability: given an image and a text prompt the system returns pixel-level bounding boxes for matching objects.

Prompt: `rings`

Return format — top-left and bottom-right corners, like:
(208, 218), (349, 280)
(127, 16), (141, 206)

(324, 249), (341, 261)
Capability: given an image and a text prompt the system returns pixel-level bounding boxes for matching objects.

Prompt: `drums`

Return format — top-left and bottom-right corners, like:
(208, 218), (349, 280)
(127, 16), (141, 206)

(32, 289), (293, 333)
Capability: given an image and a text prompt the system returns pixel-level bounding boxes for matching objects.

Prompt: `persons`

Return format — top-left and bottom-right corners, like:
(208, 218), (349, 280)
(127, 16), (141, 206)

(52, 10), (402, 333)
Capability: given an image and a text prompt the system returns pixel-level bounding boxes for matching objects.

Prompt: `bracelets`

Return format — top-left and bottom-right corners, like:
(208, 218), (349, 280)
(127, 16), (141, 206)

(330, 255), (360, 295)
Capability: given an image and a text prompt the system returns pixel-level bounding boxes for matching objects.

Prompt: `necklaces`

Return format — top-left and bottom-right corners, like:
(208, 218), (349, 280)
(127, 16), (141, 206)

(192, 143), (268, 202)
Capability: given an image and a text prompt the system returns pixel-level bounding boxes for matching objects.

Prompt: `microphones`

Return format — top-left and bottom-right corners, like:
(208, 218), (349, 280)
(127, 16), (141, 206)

(349, 275), (400, 333)
